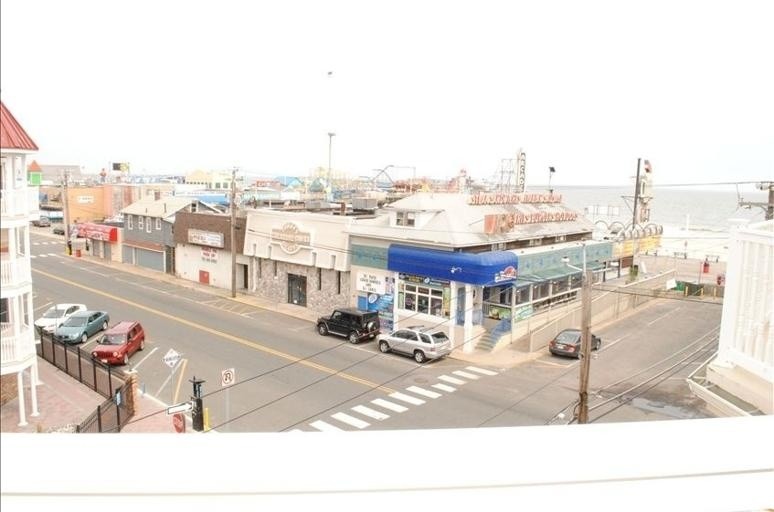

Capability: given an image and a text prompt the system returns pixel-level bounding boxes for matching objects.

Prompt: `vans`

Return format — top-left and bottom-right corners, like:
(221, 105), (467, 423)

(30, 215), (51, 229)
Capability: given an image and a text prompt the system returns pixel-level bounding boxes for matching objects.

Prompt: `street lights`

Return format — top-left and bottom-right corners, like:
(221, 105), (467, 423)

(547, 164), (556, 187)
(326, 131), (336, 176)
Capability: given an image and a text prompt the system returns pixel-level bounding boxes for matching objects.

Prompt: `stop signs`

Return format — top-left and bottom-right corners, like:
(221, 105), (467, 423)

(171, 413), (184, 433)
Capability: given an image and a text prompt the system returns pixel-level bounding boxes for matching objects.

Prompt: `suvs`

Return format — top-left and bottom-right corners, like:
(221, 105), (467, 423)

(313, 306), (381, 346)
(89, 317), (147, 368)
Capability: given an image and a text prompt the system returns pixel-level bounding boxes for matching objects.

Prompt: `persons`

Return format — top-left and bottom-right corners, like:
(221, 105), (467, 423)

(68, 221), (77, 233)
(99, 167), (106, 185)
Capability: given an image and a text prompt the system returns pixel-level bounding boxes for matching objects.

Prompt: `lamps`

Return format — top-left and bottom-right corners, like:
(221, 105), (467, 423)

(543, 281), (558, 286)
(451, 266), (462, 274)
(516, 288), (520, 293)
(532, 284), (539, 289)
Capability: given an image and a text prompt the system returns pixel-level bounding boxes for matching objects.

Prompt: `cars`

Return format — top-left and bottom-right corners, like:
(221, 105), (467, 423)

(546, 327), (602, 360)
(375, 322), (454, 364)
(52, 226), (64, 236)
(33, 301), (88, 335)
(53, 309), (111, 346)
